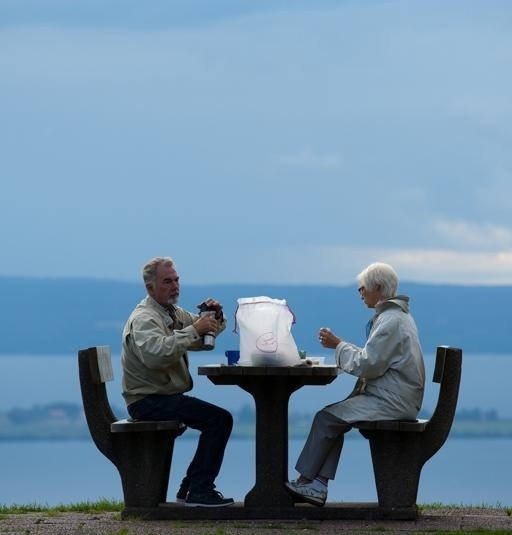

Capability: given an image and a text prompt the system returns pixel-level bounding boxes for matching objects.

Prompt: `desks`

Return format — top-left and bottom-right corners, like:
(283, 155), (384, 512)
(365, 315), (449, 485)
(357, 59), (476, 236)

(197, 363), (339, 522)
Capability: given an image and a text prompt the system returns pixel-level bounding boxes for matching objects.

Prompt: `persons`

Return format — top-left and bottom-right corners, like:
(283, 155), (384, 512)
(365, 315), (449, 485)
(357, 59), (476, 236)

(121, 256), (234, 507)
(284, 262), (425, 507)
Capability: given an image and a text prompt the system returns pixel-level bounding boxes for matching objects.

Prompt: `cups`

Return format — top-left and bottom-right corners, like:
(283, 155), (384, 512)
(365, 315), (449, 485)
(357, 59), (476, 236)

(226, 350), (240, 365)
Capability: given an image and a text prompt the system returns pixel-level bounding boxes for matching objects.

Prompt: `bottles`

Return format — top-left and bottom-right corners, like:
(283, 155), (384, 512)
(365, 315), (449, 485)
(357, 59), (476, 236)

(199, 304), (216, 351)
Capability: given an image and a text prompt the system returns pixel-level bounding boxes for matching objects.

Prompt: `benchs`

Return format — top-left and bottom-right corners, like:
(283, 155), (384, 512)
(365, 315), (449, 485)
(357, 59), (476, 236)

(77, 343), (187, 521)
(351, 344), (462, 522)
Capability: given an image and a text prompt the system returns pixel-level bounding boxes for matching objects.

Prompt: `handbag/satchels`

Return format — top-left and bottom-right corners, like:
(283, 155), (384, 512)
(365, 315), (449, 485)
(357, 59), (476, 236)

(234, 295), (302, 367)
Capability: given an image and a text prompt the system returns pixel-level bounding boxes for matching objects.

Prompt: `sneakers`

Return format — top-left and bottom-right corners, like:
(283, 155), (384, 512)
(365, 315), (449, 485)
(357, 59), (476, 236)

(176, 487), (188, 503)
(284, 479), (328, 507)
(184, 489), (234, 508)
